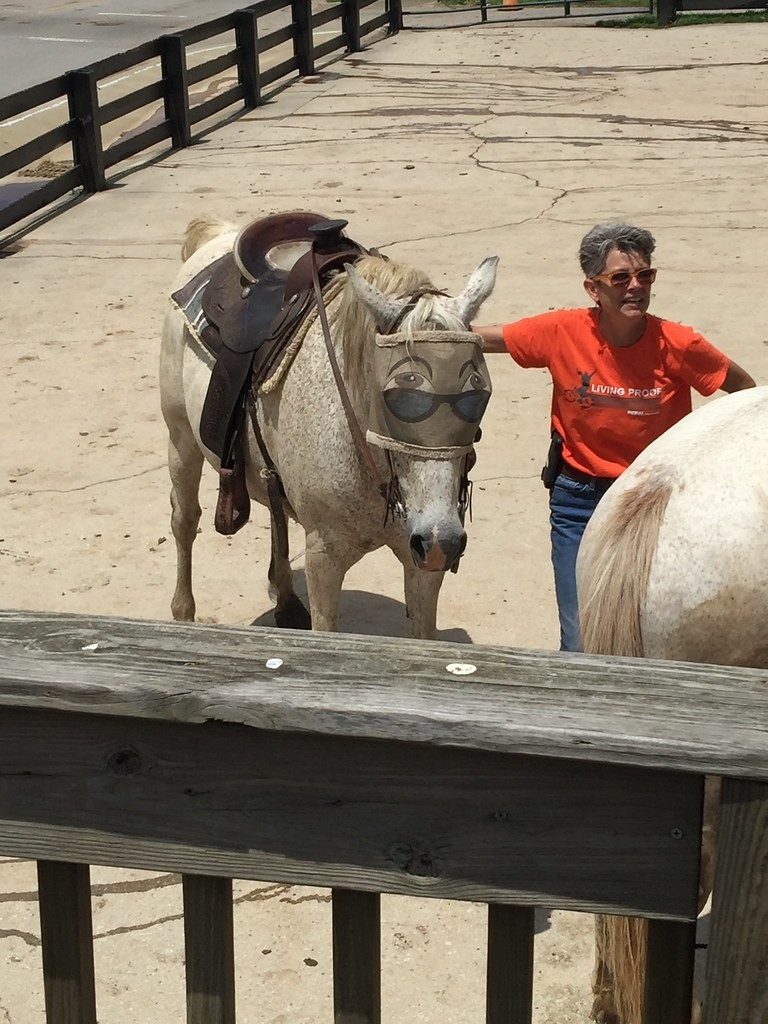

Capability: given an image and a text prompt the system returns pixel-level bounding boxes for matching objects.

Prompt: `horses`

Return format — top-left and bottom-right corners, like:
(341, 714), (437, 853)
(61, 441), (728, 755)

(159, 212), (500, 638)
(575, 385), (768, 1023)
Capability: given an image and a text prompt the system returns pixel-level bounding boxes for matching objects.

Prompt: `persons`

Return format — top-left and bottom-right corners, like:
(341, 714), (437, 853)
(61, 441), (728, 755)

(468, 217), (758, 651)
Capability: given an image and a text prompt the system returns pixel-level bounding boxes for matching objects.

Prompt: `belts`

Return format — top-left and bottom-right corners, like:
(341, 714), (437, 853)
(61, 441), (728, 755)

(560, 458), (616, 489)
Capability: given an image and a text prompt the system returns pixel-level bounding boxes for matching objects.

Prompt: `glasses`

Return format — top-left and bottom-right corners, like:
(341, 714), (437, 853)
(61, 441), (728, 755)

(592, 268), (657, 290)
